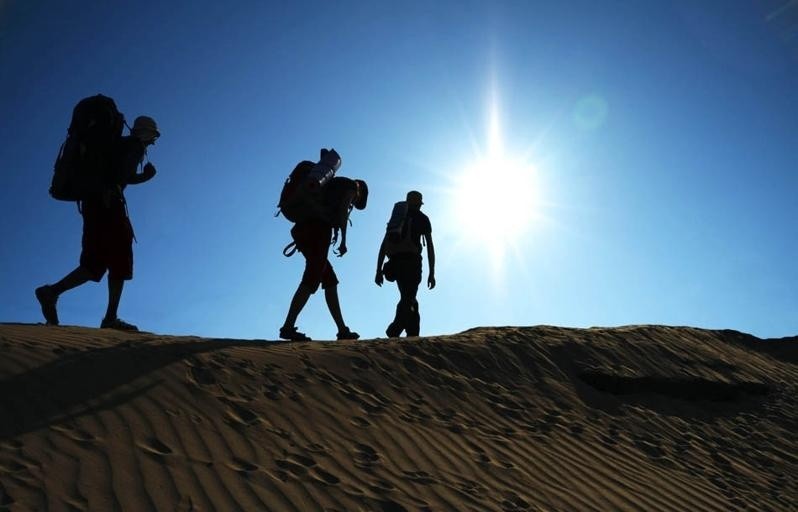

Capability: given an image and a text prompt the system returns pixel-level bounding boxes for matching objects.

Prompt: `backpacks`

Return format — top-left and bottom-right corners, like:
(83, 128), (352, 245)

(385, 201), (411, 260)
(271, 147), (341, 223)
(46, 93), (148, 202)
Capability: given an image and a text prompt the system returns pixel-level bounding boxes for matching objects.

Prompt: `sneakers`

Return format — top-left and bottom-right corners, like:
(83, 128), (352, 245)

(336, 328), (360, 339)
(34, 283), (59, 325)
(280, 326), (313, 341)
(100, 316), (138, 332)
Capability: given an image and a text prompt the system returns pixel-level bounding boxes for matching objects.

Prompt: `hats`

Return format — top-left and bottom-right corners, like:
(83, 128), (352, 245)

(130, 115), (160, 138)
(407, 190), (424, 205)
(356, 179), (369, 209)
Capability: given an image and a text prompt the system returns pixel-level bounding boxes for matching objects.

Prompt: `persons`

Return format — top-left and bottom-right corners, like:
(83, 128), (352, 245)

(279, 177), (369, 341)
(373, 190), (436, 338)
(35, 115), (162, 330)
